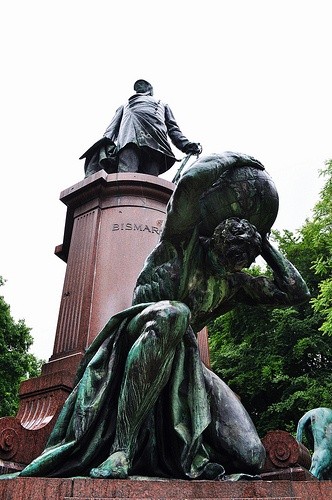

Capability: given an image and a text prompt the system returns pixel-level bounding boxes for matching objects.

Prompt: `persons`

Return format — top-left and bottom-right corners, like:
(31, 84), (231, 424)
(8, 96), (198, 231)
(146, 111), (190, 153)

(104, 79), (200, 178)
(88, 216), (312, 478)
